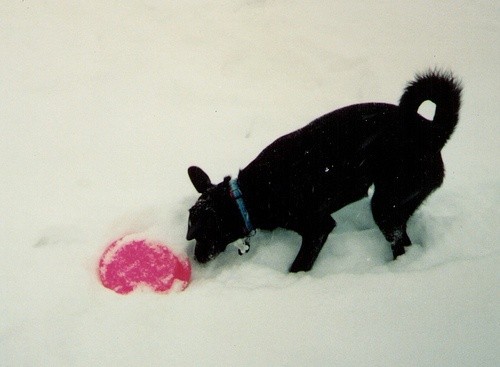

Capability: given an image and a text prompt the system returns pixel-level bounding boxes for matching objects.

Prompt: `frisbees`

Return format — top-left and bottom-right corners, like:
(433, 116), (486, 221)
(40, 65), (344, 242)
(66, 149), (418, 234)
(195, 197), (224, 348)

(98, 236), (191, 295)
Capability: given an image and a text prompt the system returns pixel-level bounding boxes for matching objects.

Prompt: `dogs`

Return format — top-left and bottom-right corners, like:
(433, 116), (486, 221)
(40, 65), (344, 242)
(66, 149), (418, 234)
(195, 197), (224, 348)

(186, 64), (465, 274)
